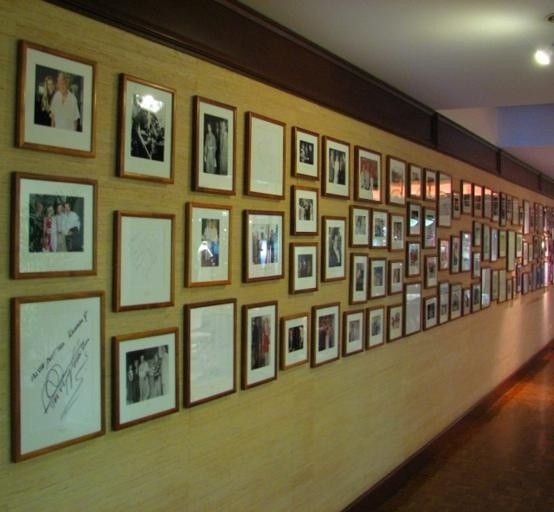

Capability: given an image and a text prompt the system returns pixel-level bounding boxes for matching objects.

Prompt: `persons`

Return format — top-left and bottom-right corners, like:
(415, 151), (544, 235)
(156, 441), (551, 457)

(203, 221), (217, 260)
(128, 346), (169, 402)
(329, 234), (341, 267)
(40, 71), (81, 131)
(32, 199), (80, 251)
(252, 317), (270, 368)
(258, 229), (274, 264)
(204, 121), (228, 175)
(329, 149), (346, 185)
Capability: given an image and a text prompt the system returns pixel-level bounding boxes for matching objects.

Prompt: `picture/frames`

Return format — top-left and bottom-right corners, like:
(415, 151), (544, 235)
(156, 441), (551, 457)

(474, 181), (554, 314)
(8, 38), (290, 464)
(290, 127), (474, 368)
